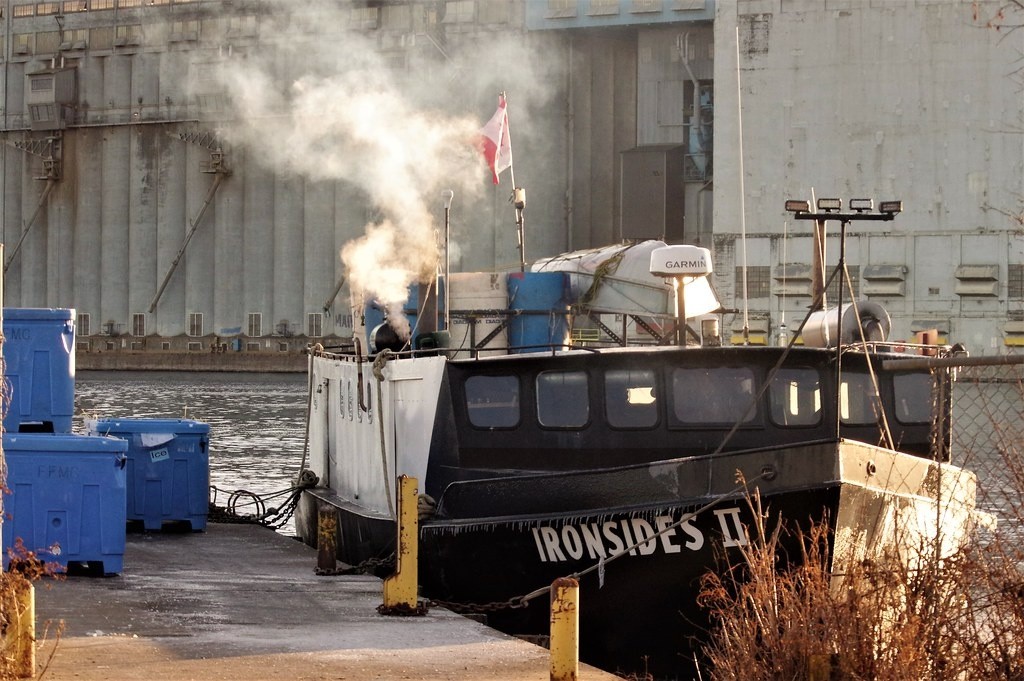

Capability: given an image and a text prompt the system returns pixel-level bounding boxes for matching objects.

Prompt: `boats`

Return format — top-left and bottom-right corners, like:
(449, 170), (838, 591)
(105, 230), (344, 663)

(288, 186), (953, 681)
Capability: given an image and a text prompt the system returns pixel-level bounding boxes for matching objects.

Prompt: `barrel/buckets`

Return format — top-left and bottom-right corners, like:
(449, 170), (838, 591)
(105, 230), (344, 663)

(447, 272), (571, 360)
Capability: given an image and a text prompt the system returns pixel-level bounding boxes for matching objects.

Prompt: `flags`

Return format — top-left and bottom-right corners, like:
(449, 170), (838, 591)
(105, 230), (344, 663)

(474, 101), (512, 184)
